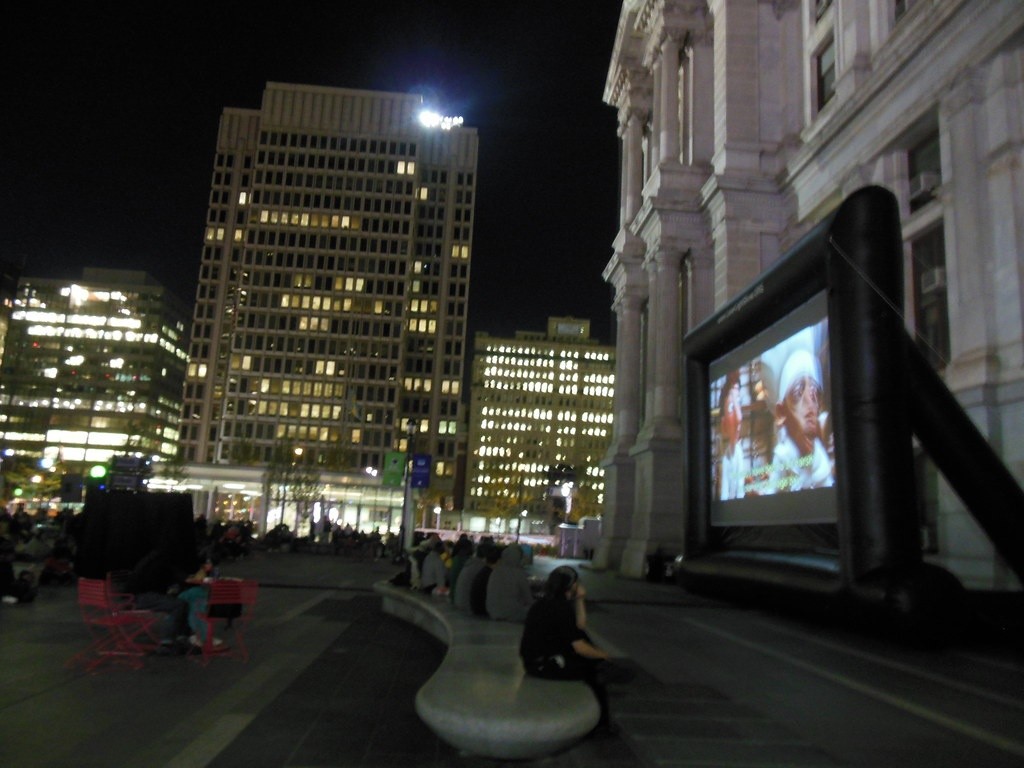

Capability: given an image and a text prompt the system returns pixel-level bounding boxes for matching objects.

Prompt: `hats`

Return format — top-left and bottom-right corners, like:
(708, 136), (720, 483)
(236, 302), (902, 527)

(223, 527), (238, 540)
(779, 350), (823, 404)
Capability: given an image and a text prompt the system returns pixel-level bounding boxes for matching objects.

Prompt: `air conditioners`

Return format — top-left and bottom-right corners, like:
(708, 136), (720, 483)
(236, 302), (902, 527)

(816, 0), (829, 19)
(921, 267), (946, 295)
(922, 527), (934, 551)
(908, 172), (938, 206)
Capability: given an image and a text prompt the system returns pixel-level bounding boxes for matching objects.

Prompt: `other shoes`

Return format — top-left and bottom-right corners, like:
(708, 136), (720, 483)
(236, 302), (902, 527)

(600, 662), (636, 685)
(589, 722), (620, 741)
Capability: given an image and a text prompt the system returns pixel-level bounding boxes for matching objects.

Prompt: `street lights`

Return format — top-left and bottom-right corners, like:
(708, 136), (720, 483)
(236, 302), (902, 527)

(415, 105), (464, 531)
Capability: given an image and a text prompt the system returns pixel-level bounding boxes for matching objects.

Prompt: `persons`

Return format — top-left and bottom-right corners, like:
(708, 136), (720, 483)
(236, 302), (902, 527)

(486, 546), (537, 623)
(193, 514), (405, 575)
(520, 568), (635, 743)
(2, 505), (81, 604)
(403, 533), (505, 621)
(135, 555), (205, 656)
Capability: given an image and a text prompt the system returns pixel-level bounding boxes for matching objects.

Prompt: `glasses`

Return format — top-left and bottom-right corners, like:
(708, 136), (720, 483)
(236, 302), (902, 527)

(787, 380), (818, 405)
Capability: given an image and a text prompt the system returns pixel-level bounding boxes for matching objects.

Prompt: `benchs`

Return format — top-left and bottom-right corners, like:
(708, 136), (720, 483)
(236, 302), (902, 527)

(372, 579), (599, 763)
(250, 541), (352, 556)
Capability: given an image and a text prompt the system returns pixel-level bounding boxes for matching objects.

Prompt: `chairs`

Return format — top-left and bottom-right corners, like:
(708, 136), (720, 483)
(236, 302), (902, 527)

(172, 578), (261, 668)
(77, 573), (164, 675)
